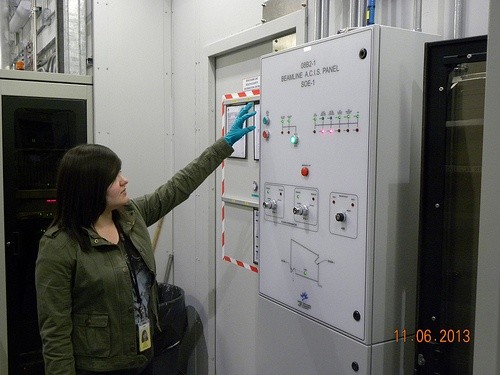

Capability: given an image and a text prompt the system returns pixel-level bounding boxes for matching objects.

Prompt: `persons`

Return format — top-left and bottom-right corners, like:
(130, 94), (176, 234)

(35, 100), (257, 375)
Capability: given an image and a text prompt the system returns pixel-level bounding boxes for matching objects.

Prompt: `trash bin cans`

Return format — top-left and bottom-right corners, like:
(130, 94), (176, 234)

(156, 283), (190, 375)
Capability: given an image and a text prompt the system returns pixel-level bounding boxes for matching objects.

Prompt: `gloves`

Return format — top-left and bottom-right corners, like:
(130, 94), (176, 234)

(223, 102), (257, 146)
